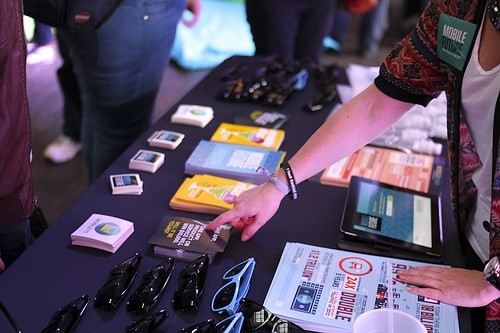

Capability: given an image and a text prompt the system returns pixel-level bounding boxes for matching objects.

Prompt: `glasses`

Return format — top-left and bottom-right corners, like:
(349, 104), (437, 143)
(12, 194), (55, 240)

(41, 294), (90, 333)
(124, 309), (169, 333)
(125, 256), (175, 315)
(171, 254), (209, 312)
(216, 53), (353, 113)
(236, 298), (306, 333)
(94, 252), (143, 313)
(211, 257), (256, 315)
(215, 312), (244, 333)
(177, 318), (215, 333)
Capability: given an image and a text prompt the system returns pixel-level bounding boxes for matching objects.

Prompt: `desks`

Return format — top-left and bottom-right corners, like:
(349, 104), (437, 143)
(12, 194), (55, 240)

(0, 55), (467, 332)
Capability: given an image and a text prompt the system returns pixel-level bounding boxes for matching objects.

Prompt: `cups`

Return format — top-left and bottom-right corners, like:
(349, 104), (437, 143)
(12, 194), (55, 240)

(353, 309), (427, 333)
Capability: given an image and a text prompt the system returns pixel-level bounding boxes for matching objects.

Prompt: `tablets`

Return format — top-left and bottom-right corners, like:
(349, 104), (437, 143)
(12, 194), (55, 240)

(339, 175), (442, 257)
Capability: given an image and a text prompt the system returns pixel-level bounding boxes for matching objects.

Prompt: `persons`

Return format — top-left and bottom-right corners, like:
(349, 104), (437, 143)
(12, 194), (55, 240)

(326, 0), (391, 57)
(243, 0), (337, 64)
(40, 27), (83, 165)
(0, 0), (35, 276)
(205, 0), (500, 333)
(56, 0), (202, 186)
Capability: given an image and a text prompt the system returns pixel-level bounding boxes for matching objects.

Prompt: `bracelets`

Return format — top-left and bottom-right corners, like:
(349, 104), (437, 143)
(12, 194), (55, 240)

(280, 161), (299, 200)
(256, 166), (291, 194)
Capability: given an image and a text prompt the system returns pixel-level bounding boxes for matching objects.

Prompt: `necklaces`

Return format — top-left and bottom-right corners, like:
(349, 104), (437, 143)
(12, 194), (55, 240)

(486, 0), (500, 31)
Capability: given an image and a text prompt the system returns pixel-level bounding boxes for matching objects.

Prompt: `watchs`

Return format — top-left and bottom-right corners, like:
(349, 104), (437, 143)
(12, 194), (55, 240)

(484, 256), (500, 293)
